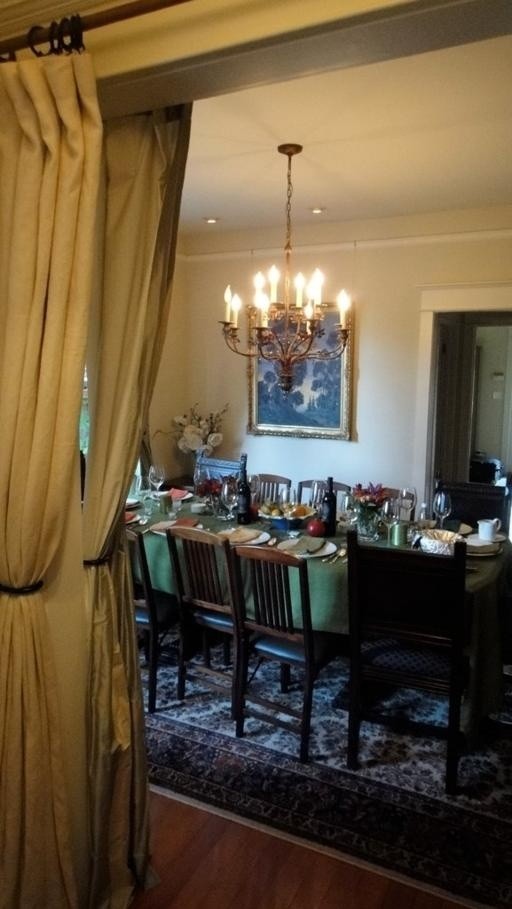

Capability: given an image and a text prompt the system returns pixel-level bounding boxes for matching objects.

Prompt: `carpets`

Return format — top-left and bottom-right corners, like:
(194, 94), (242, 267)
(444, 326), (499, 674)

(137, 621), (511, 908)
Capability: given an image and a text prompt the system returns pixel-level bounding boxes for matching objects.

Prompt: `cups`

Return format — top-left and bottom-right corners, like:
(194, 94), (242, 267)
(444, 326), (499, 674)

(125, 512), (141, 524)
(310, 480), (326, 512)
(477, 518), (502, 540)
(340, 496), (361, 525)
(248, 472), (260, 505)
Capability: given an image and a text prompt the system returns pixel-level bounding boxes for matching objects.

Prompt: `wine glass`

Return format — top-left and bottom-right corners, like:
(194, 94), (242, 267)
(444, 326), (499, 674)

(193, 468), (236, 521)
(149, 464), (166, 507)
(433, 492), (452, 530)
(398, 488), (416, 524)
(278, 487), (297, 541)
(380, 501), (399, 546)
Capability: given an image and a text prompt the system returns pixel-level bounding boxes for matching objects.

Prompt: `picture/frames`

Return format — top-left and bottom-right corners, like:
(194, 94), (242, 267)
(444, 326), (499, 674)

(248, 303), (353, 441)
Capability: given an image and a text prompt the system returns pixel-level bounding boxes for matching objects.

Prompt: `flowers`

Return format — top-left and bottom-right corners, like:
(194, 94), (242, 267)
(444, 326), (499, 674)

(172, 400), (232, 458)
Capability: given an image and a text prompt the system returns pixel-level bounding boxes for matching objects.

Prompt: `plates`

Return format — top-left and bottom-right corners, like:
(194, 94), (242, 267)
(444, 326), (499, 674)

(217, 529), (271, 546)
(466, 547), (504, 557)
(149, 520), (203, 537)
(467, 533), (506, 543)
(125, 498), (141, 510)
(277, 537), (338, 559)
(418, 520), (473, 535)
(150, 490), (194, 502)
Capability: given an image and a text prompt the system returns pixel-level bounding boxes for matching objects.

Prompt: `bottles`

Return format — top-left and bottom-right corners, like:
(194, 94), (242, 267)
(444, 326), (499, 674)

(236, 456), (251, 525)
(321, 477), (337, 536)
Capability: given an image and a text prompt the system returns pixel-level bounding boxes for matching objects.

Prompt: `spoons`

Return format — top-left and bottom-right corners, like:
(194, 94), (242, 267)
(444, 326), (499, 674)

(321, 548), (346, 564)
(268, 536), (278, 546)
(128, 518), (149, 530)
(467, 548), (501, 554)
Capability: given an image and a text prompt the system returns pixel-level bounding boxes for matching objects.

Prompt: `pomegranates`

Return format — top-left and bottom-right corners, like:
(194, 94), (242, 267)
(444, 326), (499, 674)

(307, 517), (325, 537)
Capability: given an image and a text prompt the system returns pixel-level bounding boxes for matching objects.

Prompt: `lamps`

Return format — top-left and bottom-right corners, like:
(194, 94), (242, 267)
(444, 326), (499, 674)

(219, 143), (354, 394)
